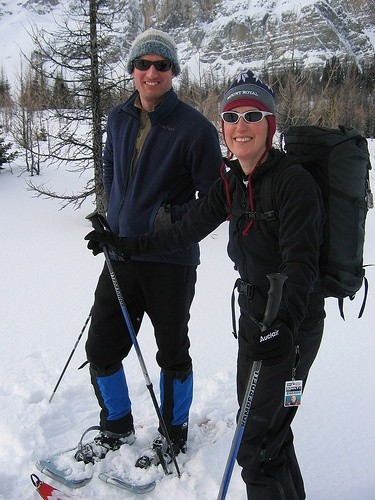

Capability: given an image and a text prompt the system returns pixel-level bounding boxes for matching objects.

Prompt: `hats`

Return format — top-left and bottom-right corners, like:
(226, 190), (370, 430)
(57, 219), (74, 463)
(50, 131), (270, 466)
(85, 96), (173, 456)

(219, 69), (277, 152)
(127, 27), (181, 76)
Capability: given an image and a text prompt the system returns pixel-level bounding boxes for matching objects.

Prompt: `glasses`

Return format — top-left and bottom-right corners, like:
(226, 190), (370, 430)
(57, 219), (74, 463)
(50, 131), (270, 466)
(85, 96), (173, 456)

(132, 59), (171, 72)
(220, 111), (273, 124)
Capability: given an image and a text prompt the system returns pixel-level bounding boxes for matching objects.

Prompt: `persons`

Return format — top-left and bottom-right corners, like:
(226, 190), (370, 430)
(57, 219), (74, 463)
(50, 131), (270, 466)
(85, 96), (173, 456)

(84, 69), (327, 500)
(74, 27), (228, 470)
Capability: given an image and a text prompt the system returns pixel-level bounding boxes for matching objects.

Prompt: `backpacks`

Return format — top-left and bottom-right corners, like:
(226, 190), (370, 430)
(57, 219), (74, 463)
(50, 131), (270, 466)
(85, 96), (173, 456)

(229, 124), (373, 301)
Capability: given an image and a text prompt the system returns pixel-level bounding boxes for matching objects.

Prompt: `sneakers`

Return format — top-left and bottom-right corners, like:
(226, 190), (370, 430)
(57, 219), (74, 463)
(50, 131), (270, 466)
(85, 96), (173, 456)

(135, 434), (187, 469)
(75, 425), (136, 464)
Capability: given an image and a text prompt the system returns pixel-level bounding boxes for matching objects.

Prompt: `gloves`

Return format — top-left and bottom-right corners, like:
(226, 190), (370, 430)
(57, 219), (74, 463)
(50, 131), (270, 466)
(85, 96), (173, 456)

(153, 204), (172, 231)
(84, 228), (128, 262)
(247, 318), (293, 366)
